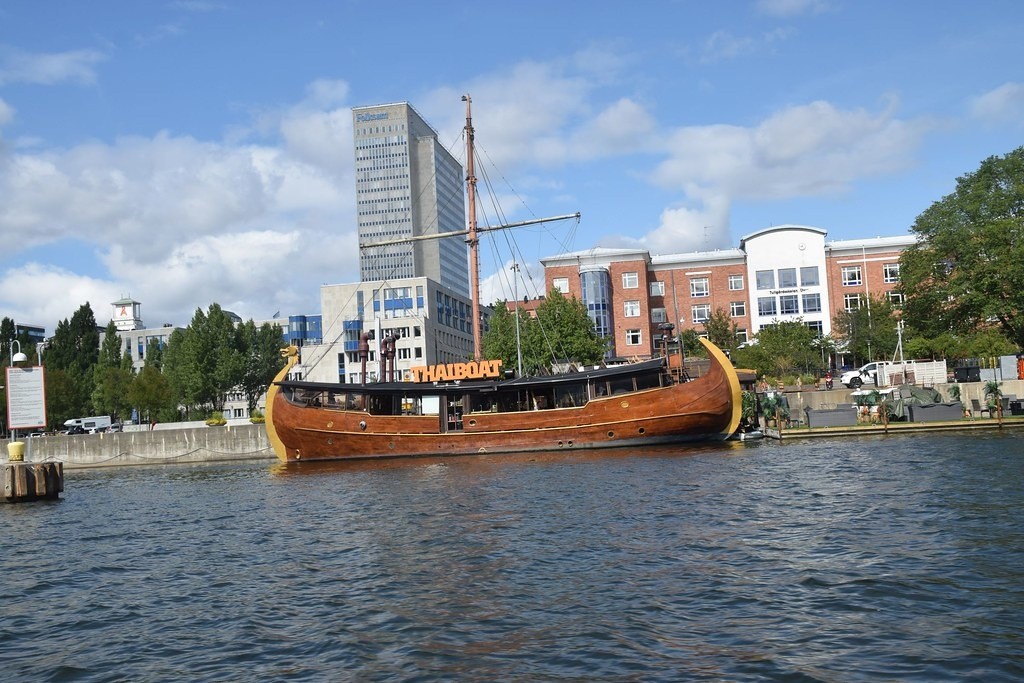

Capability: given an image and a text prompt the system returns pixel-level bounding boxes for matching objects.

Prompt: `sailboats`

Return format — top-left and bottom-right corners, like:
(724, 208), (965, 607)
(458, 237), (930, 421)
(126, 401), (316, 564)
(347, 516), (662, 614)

(264, 92), (743, 463)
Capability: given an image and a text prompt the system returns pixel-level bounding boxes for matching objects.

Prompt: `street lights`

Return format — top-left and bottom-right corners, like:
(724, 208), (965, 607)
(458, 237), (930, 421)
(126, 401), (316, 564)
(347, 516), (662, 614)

(7, 340), (28, 462)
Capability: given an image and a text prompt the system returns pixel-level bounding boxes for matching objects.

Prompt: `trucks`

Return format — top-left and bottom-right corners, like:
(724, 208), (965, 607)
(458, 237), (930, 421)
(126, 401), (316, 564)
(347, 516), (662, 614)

(64, 416), (122, 435)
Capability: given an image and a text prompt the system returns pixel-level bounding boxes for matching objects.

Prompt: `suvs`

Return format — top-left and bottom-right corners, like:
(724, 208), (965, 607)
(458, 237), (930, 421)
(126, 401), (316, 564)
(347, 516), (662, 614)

(840, 361), (893, 388)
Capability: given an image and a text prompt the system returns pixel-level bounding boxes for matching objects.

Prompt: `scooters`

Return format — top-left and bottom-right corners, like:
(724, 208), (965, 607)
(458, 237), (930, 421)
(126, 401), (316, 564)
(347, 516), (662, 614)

(825, 370), (833, 390)
